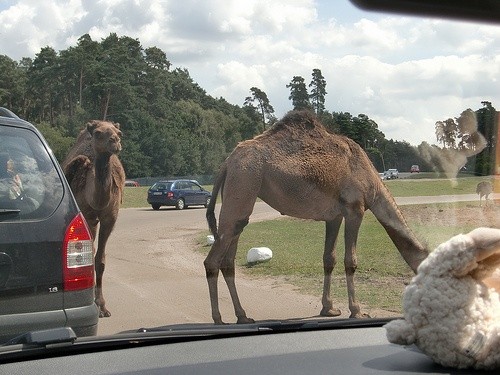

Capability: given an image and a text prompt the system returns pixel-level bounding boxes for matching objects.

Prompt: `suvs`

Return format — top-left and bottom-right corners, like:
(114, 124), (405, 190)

(0, 105), (100, 346)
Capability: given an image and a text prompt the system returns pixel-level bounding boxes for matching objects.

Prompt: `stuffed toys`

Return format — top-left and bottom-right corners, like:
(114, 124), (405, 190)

(383, 227), (500, 370)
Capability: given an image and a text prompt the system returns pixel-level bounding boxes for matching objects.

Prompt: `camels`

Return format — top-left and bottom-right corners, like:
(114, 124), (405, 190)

(63, 117), (128, 320)
(202, 106), (433, 327)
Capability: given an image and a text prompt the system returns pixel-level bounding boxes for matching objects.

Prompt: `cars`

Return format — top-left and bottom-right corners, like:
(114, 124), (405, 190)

(125, 181), (139, 187)
(411, 165), (419, 173)
(379, 169), (399, 180)
(146, 179), (212, 210)
